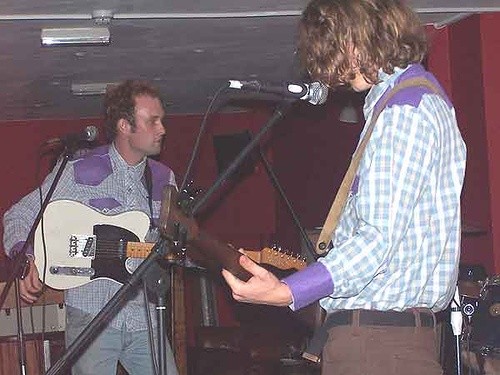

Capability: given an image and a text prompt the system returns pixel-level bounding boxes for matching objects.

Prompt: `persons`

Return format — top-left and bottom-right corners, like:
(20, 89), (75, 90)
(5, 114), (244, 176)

(222, 0), (468, 375)
(0, 78), (182, 375)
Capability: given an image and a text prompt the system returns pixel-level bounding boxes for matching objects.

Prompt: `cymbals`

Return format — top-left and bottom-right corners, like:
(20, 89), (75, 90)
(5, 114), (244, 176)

(458, 279), (480, 298)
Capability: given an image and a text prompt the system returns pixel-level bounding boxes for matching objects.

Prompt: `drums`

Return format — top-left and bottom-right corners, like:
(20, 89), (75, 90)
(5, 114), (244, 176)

(464, 273), (500, 358)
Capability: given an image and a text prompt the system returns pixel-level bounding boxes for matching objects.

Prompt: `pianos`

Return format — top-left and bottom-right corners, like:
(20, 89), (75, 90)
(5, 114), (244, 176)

(1, 304), (69, 337)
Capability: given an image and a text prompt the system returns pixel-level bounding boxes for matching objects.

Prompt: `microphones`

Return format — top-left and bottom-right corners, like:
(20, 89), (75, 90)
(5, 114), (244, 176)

(228, 80), (328, 106)
(48, 126), (99, 146)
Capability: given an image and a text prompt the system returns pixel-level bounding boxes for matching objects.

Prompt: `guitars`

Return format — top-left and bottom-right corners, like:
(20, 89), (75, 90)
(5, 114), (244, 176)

(159, 183), (326, 338)
(32, 199), (312, 290)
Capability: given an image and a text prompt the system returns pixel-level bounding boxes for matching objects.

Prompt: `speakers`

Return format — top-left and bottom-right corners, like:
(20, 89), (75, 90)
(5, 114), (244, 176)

(213, 133), (255, 177)
